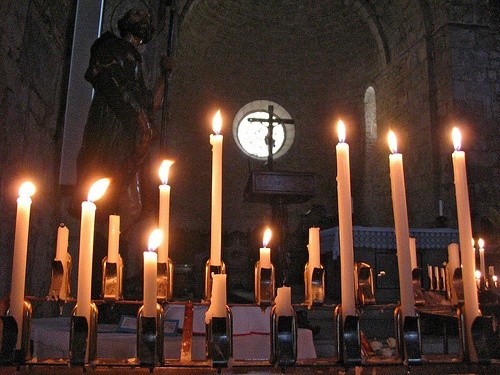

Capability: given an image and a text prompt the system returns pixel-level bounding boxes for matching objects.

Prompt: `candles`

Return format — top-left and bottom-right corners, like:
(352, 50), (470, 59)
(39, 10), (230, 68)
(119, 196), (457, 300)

(334, 118), (356, 328)
(107, 213), (120, 263)
(52, 222), (70, 301)
(308, 224), (321, 287)
(74, 176), (112, 362)
(11, 179), (37, 349)
(275, 277), (292, 316)
(143, 160), (176, 317)
(205, 271), (227, 322)
(387, 127), (417, 324)
(428, 126), (496, 361)
(208, 109), (223, 267)
(259, 226), (273, 272)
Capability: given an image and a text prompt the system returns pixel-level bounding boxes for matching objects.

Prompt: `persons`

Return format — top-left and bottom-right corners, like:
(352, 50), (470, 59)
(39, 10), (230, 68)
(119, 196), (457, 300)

(69, 8), (155, 300)
